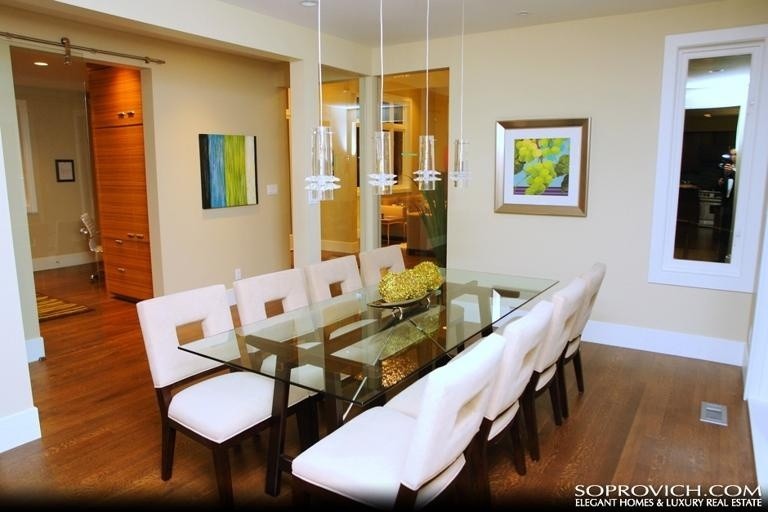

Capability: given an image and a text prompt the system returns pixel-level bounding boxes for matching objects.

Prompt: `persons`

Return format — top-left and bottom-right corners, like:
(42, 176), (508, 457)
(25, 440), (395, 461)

(716, 147), (737, 263)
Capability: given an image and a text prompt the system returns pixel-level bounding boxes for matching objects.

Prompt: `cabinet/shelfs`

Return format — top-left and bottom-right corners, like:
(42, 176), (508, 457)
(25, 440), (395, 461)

(81, 59), (152, 303)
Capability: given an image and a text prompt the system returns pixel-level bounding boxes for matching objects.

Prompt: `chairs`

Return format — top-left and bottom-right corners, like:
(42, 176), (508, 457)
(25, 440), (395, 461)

(79, 210), (103, 281)
(130, 240), (608, 512)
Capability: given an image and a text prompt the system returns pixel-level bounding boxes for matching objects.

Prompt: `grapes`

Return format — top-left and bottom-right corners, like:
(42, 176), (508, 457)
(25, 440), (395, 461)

(515, 139), (563, 195)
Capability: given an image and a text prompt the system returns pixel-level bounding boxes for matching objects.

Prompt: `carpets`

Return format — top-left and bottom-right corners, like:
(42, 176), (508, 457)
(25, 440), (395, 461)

(36, 291), (95, 324)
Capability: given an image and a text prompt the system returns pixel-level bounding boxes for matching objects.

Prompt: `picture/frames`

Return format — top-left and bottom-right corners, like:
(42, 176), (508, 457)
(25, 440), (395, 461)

(15, 99), (39, 214)
(491, 114), (592, 217)
(54, 158), (76, 182)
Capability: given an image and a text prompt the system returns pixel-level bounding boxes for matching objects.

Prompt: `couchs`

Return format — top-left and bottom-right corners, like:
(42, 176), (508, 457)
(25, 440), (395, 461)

(380, 193), (426, 239)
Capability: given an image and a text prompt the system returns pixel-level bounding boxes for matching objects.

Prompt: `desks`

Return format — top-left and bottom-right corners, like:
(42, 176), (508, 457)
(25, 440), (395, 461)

(380, 216), (406, 245)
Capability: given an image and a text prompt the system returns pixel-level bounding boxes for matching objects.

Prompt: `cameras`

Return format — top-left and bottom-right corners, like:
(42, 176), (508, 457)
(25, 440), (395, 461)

(720, 153), (732, 175)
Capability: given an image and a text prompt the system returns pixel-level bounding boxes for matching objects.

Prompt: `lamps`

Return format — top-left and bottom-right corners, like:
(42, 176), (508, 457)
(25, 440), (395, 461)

(301, 0), (339, 201)
(449, 0), (475, 187)
(413, 0), (442, 192)
(365, 0), (395, 195)
